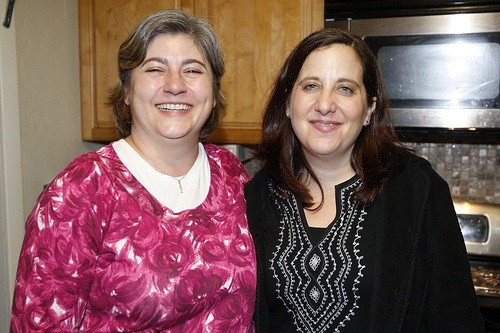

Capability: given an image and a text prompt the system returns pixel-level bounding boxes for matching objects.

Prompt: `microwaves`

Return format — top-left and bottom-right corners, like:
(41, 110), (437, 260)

(326, 3), (500, 144)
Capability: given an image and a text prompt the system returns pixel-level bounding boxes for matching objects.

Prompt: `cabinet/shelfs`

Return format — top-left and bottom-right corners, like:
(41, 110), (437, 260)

(78, 0), (324, 146)
(324, 5), (500, 130)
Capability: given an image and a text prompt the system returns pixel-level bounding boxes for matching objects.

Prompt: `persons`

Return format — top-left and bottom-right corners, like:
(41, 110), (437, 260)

(244, 29), (481, 333)
(9, 9), (257, 333)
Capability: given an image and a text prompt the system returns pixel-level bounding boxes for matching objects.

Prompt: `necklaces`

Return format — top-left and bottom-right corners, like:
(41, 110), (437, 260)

(130, 136), (200, 193)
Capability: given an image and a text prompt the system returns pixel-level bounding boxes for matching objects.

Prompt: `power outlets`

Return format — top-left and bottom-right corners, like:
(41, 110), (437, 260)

(223, 145), (242, 161)
